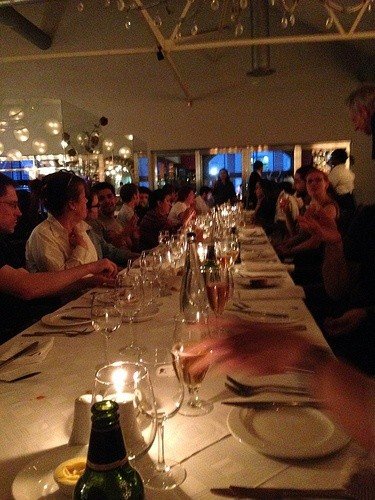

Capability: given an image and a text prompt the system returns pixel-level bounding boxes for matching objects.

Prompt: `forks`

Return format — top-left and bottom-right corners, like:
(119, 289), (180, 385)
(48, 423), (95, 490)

(24, 327), (88, 339)
(227, 374), (312, 391)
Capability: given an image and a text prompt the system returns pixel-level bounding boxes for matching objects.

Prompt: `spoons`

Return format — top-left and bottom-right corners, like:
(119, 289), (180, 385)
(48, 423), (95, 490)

(225, 382), (311, 398)
(33, 329), (95, 335)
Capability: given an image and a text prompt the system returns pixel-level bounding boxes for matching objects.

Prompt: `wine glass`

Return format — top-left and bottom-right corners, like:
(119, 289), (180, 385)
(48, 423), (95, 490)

(201, 266), (232, 341)
(91, 291), (125, 372)
(135, 348), (187, 494)
(170, 311), (214, 418)
(115, 272), (144, 356)
(91, 360), (154, 467)
(129, 231), (183, 310)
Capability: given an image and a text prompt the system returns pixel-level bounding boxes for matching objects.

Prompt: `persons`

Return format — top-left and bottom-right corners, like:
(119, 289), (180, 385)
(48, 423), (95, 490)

(0, 174), (118, 340)
(25, 170), (98, 281)
(85, 148), (375, 378)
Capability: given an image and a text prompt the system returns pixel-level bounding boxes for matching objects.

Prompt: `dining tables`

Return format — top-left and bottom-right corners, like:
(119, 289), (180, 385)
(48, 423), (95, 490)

(0, 196), (375, 500)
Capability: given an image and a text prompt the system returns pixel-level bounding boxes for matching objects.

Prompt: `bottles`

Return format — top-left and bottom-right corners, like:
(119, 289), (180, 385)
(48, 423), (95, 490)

(178, 230), (210, 319)
(74, 399), (144, 500)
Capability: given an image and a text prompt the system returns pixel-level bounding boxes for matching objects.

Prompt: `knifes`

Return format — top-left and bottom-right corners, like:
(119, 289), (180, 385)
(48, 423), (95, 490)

(220, 400), (327, 409)
(0, 340), (40, 369)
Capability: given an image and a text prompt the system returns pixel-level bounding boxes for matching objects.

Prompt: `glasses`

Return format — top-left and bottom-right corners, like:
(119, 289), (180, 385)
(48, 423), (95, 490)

(307, 176), (323, 185)
(59, 169), (75, 185)
(91, 202), (100, 208)
(98, 194), (114, 202)
(0, 201), (20, 208)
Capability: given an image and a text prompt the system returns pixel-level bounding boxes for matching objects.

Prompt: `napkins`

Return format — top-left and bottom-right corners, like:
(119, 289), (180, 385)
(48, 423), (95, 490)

(236, 286), (311, 301)
(245, 261), (297, 271)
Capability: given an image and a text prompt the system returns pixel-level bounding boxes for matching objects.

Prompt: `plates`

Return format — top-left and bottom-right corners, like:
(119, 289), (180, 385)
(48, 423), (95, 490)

(225, 389), (357, 462)
(11, 445), (88, 500)
(236, 302), (306, 323)
(42, 309), (91, 327)
